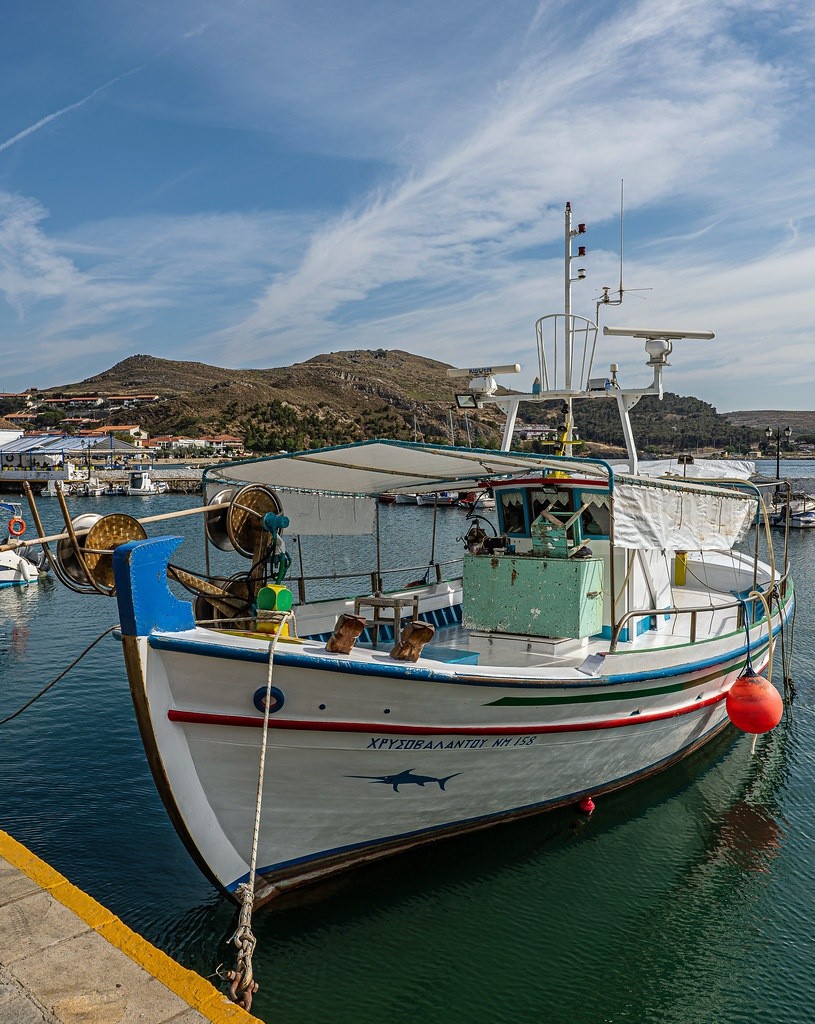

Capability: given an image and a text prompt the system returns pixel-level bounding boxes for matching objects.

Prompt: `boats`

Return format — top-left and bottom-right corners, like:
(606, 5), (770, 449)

(1, 175), (799, 940)
(748, 479), (815, 534)
(124, 470), (167, 496)
(40, 478), (73, 498)
(103, 477), (129, 495)
(1, 499), (58, 588)
(75, 477), (107, 496)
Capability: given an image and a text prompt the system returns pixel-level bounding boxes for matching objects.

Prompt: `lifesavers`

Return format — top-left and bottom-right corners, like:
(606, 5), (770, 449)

(8, 518), (26, 535)
(6, 455), (14, 462)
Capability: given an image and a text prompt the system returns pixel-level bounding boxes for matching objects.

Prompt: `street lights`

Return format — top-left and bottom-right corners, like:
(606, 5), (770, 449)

(765, 424), (792, 494)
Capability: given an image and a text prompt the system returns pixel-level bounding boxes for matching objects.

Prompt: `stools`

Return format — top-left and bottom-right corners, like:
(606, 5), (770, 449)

(354, 594), (421, 650)
(531, 499), (592, 558)
